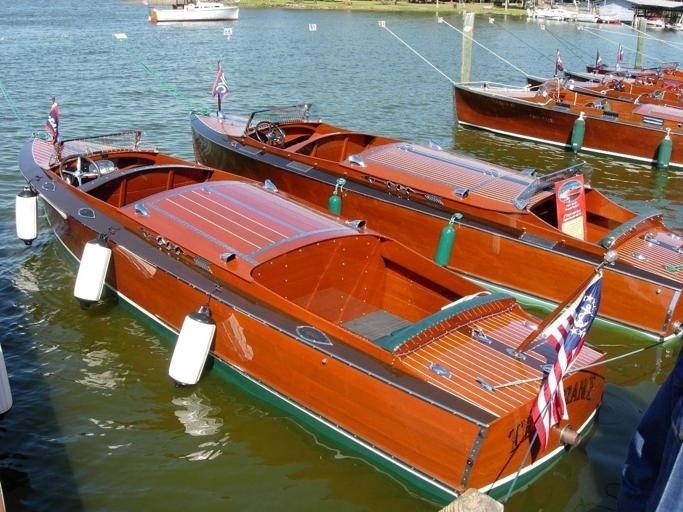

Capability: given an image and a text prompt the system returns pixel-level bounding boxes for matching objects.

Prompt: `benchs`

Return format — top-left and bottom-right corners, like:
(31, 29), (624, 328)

(372, 289), (509, 352)
(592, 208), (665, 254)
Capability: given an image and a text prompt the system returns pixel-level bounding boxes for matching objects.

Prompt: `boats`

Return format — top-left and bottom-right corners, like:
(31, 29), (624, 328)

(186, 108), (682, 347)
(149, 0), (240, 23)
(449, 63), (683, 172)
(15, 124), (606, 507)
(526, 8), (683, 33)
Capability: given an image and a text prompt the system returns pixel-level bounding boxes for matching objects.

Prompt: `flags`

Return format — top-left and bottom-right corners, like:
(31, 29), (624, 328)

(530, 275), (603, 454)
(617, 44), (624, 63)
(557, 50), (567, 72)
(596, 48), (602, 70)
(213, 66), (230, 98)
(45, 103), (59, 139)
